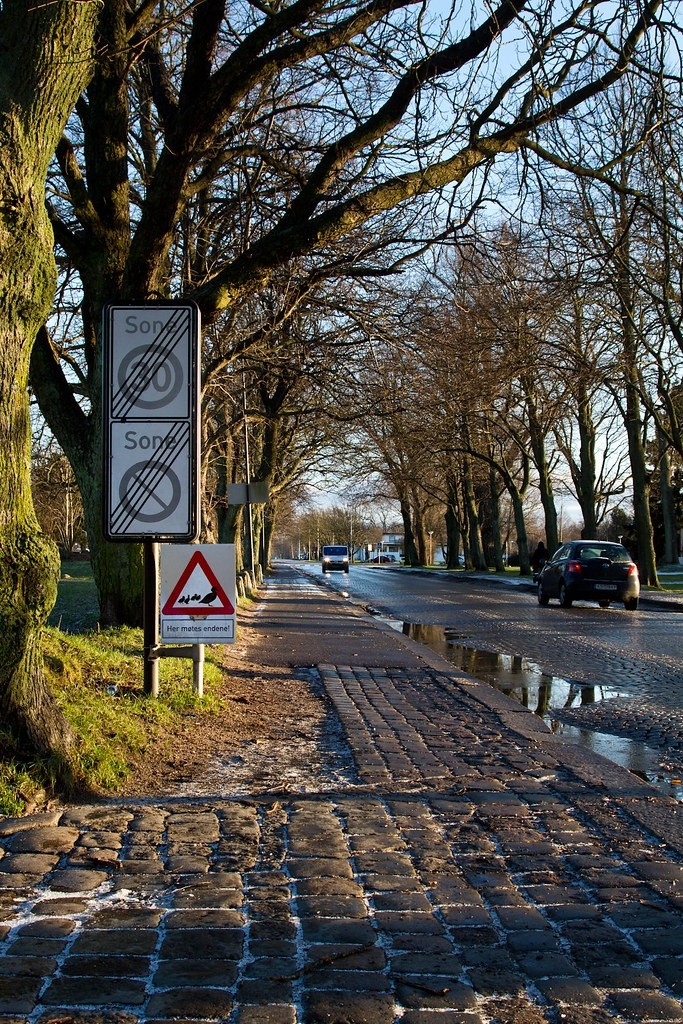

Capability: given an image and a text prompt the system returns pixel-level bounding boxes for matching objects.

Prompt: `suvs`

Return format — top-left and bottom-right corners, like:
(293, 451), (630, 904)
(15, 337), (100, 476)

(321, 544), (350, 574)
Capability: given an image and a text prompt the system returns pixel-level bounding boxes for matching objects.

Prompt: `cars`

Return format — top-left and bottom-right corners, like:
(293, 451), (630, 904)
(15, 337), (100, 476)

(371, 555), (390, 563)
(294, 554), (306, 560)
(535, 539), (640, 611)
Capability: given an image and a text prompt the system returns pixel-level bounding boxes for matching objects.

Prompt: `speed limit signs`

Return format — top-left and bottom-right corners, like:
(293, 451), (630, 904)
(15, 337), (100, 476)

(103, 306), (193, 422)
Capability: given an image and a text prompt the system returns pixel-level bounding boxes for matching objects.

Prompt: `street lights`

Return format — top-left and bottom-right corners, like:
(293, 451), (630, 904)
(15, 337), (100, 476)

(427, 531), (435, 565)
(459, 554), (466, 566)
